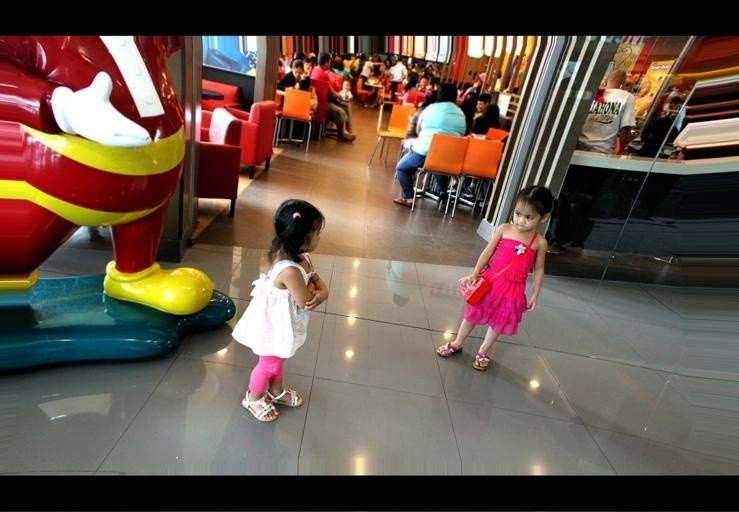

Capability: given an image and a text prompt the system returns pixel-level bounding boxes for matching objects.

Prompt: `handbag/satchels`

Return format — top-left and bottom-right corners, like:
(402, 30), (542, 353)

(459, 275), (492, 306)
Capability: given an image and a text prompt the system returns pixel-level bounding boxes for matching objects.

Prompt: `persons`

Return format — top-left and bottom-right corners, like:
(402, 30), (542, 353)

(230, 198), (330, 423)
(545, 71), (687, 254)
(278, 51), (502, 209)
(436, 185), (560, 371)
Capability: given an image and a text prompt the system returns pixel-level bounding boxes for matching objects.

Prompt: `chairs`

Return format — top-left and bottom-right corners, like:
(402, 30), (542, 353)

(194, 51), (621, 222)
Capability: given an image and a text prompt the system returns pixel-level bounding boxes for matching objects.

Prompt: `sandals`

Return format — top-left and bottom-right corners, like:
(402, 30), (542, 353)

(472, 352), (492, 371)
(241, 388), (279, 423)
(264, 388), (305, 408)
(437, 342), (463, 358)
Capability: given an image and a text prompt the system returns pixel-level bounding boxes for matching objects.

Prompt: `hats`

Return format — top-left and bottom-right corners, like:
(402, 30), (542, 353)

(478, 72), (486, 82)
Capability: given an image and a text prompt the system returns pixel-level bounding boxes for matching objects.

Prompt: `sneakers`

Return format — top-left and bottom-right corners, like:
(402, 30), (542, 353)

(338, 133), (356, 141)
(393, 198), (416, 208)
